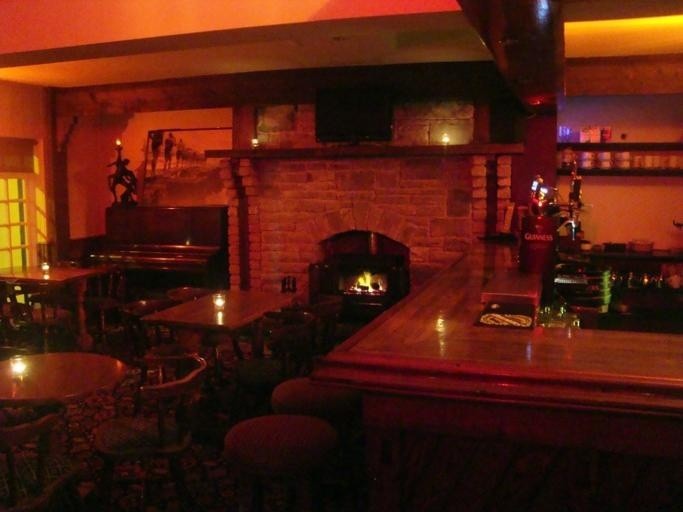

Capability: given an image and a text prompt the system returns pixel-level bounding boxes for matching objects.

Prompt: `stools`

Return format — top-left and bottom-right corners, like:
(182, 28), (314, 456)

(270, 376), (361, 511)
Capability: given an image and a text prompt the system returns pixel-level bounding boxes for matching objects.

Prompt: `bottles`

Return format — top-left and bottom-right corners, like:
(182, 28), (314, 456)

(627, 272), (663, 289)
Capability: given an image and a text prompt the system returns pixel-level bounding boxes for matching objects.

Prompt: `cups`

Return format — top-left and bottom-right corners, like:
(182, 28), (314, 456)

(578, 151), (683, 169)
(600, 126), (612, 144)
(211, 293), (226, 311)
(559, 126), (573, 142)
(9, 353), (29, 381)
(40, 262), (49, 274)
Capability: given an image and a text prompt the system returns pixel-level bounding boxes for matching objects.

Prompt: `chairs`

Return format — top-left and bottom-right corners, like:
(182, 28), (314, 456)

(0, 263), (343, 511)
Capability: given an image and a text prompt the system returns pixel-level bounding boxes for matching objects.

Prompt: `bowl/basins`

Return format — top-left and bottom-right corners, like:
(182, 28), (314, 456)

(631, 238), (654, 253)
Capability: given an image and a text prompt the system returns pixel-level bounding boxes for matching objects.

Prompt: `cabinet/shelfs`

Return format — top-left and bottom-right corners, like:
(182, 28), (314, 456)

(555, 142), (682, 177)
(580, 248), (682, 322)
